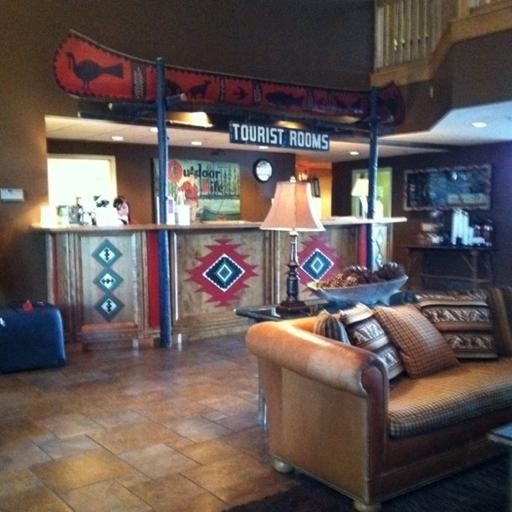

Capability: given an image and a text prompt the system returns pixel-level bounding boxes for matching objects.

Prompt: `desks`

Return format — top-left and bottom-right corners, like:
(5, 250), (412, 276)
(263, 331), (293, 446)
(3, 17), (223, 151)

(233, 297), (352, 427)
(399, 242), (496, 294)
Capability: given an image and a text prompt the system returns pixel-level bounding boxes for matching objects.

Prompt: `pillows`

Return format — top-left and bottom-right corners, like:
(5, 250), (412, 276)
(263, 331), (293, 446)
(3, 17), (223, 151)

(313, 309), (352, 345)
(414, 290), (500, 361)
(339, 303), (406, 382)
(371, 303), (459, 378)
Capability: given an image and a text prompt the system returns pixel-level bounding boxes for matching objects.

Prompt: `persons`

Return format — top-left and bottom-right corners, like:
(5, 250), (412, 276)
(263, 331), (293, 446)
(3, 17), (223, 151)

(113, 194), (142, 225)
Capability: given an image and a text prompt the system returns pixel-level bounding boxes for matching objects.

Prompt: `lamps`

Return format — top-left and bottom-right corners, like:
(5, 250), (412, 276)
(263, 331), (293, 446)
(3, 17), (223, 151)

(258, 181), (327, 315)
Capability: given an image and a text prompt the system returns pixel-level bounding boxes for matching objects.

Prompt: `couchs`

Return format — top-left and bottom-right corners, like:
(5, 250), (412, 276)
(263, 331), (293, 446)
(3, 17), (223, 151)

(244, 311), (512, 511)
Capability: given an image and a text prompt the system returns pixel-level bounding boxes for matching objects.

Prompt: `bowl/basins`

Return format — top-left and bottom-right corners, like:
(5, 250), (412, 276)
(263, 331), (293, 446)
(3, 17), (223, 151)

(307, 275), (409, 303)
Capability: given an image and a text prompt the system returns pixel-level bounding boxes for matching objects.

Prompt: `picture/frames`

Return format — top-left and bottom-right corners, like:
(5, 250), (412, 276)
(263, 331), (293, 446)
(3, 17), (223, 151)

(402, 165), (492, 212)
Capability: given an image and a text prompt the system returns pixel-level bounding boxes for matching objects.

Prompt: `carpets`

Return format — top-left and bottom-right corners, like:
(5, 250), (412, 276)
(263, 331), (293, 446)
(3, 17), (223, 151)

(219, 448), (512, 512)
